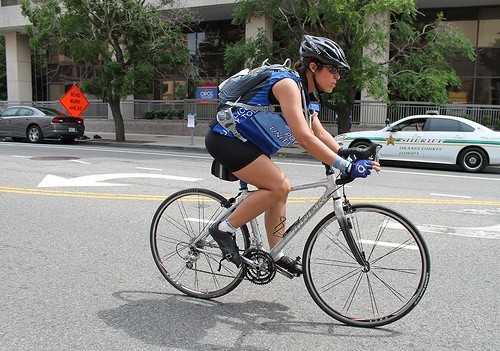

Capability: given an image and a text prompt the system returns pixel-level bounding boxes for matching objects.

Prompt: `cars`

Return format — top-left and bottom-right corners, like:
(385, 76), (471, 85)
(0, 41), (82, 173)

(0, 106), (86, 143)
(333, 109), (500, 173)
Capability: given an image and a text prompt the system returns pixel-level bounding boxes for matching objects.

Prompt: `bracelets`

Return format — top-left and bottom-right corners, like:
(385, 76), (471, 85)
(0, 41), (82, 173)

(333, 157), (343, 168)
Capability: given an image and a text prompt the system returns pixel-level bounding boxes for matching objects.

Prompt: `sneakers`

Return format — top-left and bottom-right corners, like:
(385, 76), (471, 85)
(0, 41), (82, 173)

(209, 221), (241, 266)
(275, 255), (303, 274)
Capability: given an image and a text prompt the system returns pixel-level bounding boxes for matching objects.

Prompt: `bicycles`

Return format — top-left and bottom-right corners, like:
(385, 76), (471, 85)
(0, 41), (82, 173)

(148, 143), (430, 329)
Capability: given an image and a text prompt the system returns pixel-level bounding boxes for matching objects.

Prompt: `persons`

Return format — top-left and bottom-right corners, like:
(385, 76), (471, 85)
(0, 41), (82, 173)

(204, 35), (381, 277)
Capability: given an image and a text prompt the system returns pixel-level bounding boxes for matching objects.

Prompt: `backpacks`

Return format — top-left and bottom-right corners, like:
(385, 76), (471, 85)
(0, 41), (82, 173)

(217, 58), (301, 114)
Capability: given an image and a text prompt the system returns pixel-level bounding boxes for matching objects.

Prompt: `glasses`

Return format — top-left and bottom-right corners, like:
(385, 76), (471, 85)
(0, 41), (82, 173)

(320, 64), (345, 76)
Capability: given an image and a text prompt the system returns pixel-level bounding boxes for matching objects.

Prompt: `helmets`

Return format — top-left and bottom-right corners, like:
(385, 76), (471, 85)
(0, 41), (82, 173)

(300, 35), (350, 70)
